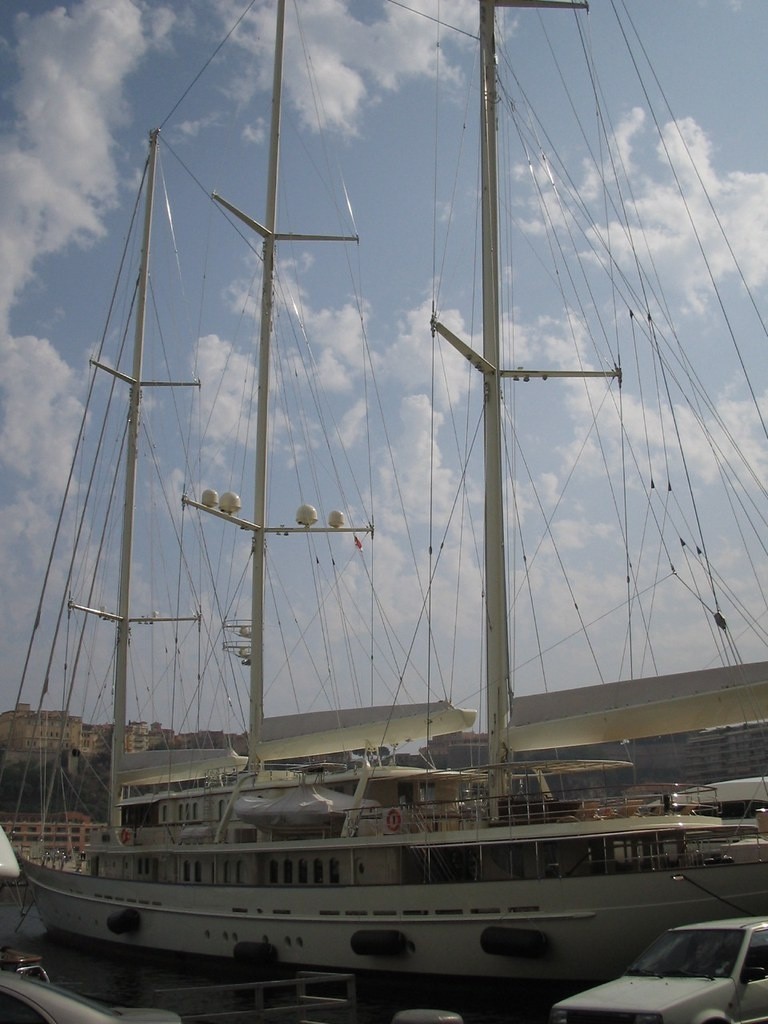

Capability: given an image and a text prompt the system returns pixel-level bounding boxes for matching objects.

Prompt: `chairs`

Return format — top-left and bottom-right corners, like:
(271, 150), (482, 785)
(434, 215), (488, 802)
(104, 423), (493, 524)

(556, 798), (700, 822)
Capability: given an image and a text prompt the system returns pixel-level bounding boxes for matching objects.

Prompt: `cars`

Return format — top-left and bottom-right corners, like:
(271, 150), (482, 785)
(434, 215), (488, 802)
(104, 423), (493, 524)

(550, 915), (768, 1024)
(0, 970), (183, 1024)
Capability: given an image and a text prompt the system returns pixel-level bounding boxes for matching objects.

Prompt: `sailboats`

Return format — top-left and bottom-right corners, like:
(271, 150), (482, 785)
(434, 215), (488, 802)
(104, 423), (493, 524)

(0, 0), (768, 1006)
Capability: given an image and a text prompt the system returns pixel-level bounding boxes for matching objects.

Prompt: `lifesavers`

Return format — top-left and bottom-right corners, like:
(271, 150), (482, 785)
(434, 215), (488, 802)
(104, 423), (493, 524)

(386, 809), (401, 831)
(121, 828), (130, 843)
